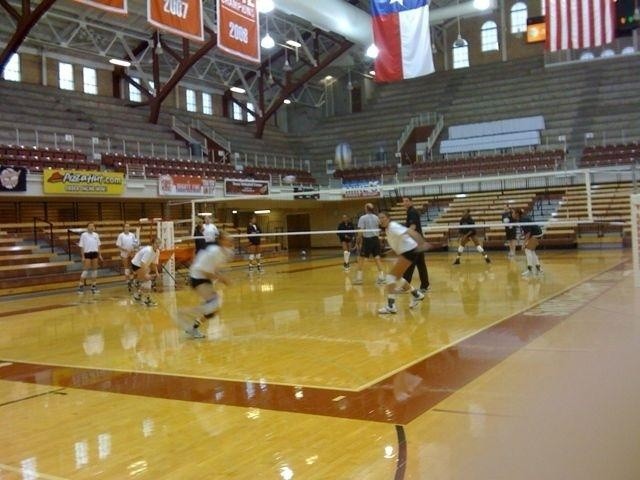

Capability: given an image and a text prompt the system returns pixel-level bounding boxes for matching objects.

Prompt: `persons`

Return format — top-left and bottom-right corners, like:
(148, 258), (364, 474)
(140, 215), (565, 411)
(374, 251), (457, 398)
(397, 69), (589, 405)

(337, 213), (356, 270)
(192, 215), (266, 272)
(184, 230), (236, 340)
(377, 211), (425, 315)
(75, 221), (104, 295)
(501, 203), (519, 257)
(353, 203), (385, 285)
(129, 237), (161, 309)
(512, 207), (544, 276)
(115, 223), (138, 289)
(402, 196), (432, 292)
(452, 208), (493, 265)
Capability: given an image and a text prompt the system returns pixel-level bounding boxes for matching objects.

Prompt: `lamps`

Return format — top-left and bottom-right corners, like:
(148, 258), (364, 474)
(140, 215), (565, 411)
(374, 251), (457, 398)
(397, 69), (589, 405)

(153, 30), (165, 57)
(109, 58), (132, 68)
(255, 10), (303, 84)
(452, 0), (469, 50)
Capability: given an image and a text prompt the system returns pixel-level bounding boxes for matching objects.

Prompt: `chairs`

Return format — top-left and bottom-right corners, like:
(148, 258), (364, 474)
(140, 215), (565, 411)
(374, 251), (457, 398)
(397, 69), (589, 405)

(332, 143), (638, 180)
(0, 144), (320, 191)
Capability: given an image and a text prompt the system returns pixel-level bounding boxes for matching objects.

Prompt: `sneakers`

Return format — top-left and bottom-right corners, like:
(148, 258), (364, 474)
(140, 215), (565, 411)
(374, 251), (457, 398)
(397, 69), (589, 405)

(410, 291), (424, 308)
(378, 303), (397, 313)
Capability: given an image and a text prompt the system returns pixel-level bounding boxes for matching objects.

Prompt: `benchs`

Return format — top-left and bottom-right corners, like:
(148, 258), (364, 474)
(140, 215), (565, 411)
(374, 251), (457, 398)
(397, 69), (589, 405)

(0, 78), (301, 166)
(302, 46), (639, 164)
(360, 182), (639, 248)
(0, 219), (281, 273)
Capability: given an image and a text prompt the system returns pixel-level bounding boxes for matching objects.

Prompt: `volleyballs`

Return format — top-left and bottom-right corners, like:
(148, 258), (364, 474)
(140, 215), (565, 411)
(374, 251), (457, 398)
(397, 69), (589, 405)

(301, 251), (307, 256)
(334, 143), (351, 170)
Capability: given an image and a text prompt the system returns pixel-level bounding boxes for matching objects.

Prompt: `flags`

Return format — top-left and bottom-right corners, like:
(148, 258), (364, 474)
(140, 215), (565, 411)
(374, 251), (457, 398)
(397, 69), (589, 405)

(216, 0), (263, 68)
(369, 0), (437, 84)
(544, 0), (617, 54)
(146, 0), (208, 47)
(68, 0), (130, 19)
